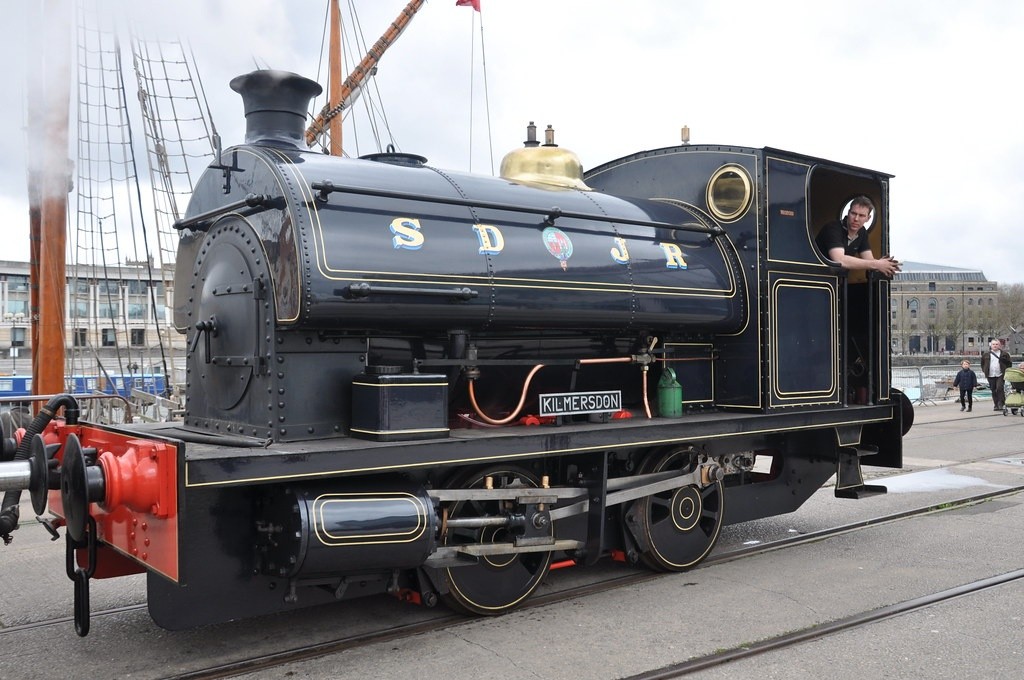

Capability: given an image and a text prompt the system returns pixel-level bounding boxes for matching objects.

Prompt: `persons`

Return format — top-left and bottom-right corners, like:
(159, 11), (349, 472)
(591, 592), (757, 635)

(816, 196), (903, 278)
(953, 360), (977, 412)
(980, 339), (1012, 410)
(1018, 362), (1024, 373)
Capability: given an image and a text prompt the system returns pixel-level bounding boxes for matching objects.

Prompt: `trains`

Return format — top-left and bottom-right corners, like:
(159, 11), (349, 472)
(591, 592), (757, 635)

(0, 70), (914, 636)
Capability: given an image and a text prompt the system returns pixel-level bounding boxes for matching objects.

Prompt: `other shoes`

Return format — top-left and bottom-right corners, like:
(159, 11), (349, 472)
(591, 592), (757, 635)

(966, 408), (972, 412)
(960, 406), (966, 411)
(994, 404), (1003, 411)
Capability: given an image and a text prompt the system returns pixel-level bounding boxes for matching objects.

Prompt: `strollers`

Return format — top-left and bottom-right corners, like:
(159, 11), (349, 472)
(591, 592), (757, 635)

(1002, 367), (1024, 416)
